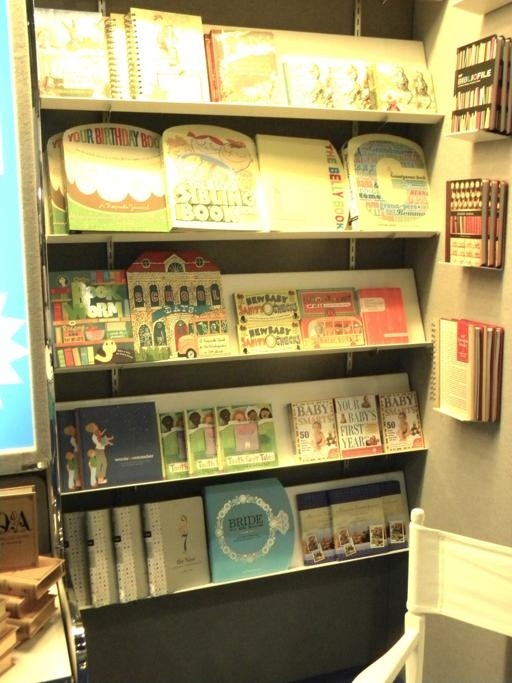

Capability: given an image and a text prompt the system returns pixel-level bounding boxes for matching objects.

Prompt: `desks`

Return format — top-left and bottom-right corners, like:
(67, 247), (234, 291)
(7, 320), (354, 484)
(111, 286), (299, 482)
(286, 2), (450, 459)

(1, 552), (72, 683)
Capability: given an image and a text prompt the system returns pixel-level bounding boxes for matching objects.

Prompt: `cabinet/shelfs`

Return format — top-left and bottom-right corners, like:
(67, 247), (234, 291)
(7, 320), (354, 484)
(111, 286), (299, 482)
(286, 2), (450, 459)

(430, 0), (510, 423)
(38, 97), (445, 611)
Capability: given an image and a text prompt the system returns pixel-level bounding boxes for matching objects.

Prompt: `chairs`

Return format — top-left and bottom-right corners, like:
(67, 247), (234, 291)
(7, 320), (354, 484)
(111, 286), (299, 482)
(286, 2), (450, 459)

(350, 509), (512, 682)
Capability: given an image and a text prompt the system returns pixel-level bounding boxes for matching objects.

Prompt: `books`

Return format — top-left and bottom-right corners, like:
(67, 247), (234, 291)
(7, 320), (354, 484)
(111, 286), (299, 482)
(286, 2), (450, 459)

(63, 478), (409, 609)
(55, 391), (429, 492)
(41, 121), (434, 236)
(47, 249), (428, 369)
(450, 32), (510, 135)
(445, 178), (507, 268)
(36, 5), (439, 113)
(437, 318), (502, 423)
